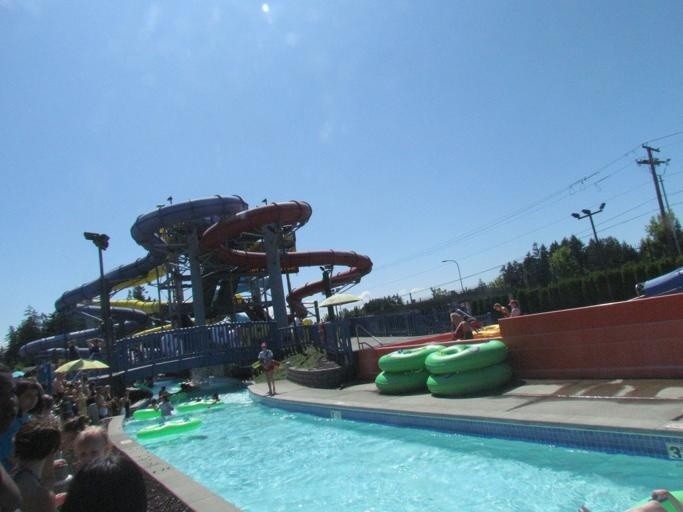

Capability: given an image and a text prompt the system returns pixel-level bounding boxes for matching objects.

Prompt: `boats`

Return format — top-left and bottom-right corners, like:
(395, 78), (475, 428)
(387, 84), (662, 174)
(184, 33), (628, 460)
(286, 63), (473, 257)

(634, 489), (683, 512)
(472, 324), (501, 338)
(374, 339), (513, 399)
(133, 392), (222, 440)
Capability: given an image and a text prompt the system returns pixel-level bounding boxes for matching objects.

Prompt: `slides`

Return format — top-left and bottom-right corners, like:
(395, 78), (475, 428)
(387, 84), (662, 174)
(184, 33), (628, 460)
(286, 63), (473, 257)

(17, 197), (372, 384)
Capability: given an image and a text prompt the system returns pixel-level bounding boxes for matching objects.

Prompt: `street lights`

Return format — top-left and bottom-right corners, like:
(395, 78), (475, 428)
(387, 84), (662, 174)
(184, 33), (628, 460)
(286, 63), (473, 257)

(84, 232), (117, 417)
(442, 259), (465, 294)
(570, 202), (607, 254)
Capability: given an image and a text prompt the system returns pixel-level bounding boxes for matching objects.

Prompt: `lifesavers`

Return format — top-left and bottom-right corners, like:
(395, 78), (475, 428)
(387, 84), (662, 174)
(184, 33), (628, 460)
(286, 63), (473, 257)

(376, 371), (428, 394)
(134, 399), (224, 439)
(426, 363), (515, 396)
(425, 340), (506, 371)
(377, 344), (445, 371)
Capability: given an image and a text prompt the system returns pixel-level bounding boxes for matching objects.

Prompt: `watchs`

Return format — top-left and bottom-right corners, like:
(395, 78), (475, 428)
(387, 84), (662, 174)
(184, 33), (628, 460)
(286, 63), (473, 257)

(492, 302), (510, 317)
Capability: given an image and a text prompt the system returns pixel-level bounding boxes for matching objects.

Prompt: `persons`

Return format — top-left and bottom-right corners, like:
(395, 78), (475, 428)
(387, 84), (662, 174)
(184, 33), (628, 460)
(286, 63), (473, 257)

(0, 339), (220, 512)
(578, 488), (683, 511)
(508, 299), (521, 316)
(257, 341), (276, 396)
(453, 315), (473, 339)
